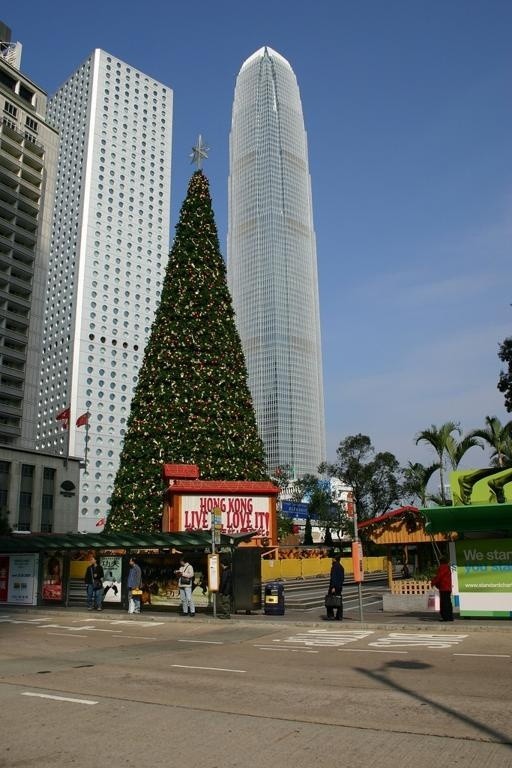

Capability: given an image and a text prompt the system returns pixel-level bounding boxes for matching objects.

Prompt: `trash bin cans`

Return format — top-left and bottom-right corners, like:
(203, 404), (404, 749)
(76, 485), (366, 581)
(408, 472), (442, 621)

(264, 583), (285, 615)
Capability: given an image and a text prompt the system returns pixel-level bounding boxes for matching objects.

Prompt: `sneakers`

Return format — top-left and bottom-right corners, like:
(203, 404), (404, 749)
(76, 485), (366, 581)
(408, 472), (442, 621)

(88, 608), (92, 611)
(182, 613), (195, 616)
(323, 616), (342, 621)
(97, 608), (101, 610)
(438, 617), (453, 621)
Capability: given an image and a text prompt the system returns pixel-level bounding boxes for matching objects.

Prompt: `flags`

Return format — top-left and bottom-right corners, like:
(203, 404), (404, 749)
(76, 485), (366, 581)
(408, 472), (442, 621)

(56, 408), (70, 421)
(76, 412), (87, 427)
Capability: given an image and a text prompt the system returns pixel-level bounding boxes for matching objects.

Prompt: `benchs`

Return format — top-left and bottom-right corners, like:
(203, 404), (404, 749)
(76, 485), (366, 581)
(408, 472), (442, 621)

(66, 578), (89, 608)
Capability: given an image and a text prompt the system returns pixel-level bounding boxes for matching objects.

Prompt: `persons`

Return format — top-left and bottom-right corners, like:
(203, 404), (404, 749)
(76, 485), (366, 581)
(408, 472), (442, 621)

(127, 556), (143, 613)
(320, 553), (345, 621)
(173, 557), (197, 617)
(218, 559), (235, 620)
(430, 553), (454, 622)
(84, 557), (103, 611)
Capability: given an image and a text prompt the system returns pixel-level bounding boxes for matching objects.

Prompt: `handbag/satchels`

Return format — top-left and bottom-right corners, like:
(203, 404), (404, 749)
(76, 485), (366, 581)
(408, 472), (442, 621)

(93, 580), (102, 590)
(424, 592), (440, 611)
(325, 595), (342, 607)
(181, 576), (190, 584)
